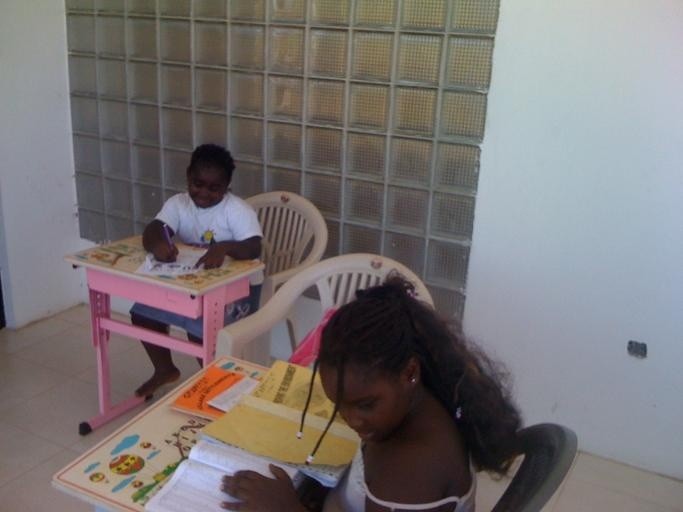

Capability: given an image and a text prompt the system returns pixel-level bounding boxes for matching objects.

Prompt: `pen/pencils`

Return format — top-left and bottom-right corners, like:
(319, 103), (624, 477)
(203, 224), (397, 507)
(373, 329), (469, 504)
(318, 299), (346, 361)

(162, 223), (173, 262)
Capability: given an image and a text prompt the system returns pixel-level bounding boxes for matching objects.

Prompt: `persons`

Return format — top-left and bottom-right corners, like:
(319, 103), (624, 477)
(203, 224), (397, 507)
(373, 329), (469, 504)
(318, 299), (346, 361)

(130, 143), (263, 397)
(218, 268), (520, 511)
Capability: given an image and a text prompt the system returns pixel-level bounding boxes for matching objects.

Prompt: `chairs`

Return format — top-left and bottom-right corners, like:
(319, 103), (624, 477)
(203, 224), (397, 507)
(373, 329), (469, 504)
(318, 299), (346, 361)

(493, 422), (578, 512)
(217, 252), (434, 368)
(245, 190), (329, 351)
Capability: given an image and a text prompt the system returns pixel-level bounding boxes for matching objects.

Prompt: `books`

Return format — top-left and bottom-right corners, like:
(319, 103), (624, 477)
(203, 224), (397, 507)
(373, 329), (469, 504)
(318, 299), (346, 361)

(144, 360), (361, 512)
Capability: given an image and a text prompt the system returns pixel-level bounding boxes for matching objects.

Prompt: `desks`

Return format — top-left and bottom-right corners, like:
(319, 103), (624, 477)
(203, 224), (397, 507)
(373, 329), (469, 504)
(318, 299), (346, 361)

(61, 234), (267, 436)
(47, 359), (356, 511)
